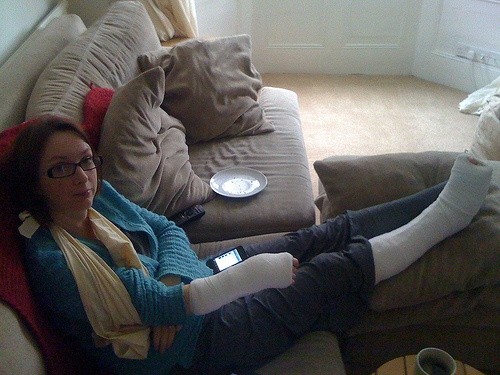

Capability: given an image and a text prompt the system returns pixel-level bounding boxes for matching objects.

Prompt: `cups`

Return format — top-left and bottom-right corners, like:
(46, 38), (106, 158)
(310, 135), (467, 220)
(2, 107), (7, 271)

(414, 347), (457, 375)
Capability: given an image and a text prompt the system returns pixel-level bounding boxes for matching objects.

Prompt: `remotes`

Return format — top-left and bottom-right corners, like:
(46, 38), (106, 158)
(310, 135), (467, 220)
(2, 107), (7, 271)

(168, 204), (205, 227)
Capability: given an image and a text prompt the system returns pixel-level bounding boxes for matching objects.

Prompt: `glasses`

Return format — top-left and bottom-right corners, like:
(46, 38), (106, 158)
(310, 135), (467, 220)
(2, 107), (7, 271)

(39, 155), (103, 178)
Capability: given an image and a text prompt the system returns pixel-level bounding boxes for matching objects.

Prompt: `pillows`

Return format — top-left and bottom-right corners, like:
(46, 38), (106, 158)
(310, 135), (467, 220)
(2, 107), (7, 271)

(313, 151), (500, 371)
(96, 66), (218, 217)
(137, 34), (276, 145)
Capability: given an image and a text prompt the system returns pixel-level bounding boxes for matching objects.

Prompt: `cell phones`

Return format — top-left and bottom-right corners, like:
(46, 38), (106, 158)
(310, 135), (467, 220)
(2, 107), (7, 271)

(206, 245), (249, 275)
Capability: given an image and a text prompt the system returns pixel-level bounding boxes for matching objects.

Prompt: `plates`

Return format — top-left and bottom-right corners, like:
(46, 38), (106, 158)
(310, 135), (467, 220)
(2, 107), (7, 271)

(210, 167), (267, 197)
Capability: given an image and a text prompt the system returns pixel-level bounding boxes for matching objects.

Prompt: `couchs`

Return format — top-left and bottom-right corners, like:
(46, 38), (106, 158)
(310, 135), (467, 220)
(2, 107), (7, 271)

(0, 0), (346, 375)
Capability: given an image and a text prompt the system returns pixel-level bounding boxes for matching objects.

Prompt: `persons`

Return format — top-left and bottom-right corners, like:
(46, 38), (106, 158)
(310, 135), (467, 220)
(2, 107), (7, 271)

(0, 115), (493, 375)
